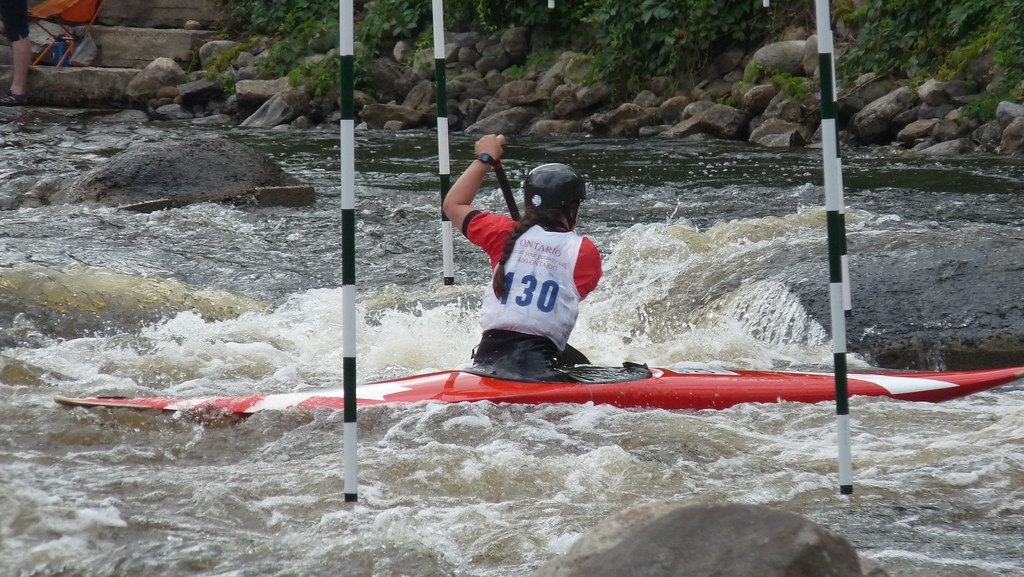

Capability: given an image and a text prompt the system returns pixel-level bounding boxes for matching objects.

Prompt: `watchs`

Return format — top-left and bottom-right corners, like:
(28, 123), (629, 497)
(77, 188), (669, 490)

(478, 152), (495, 165)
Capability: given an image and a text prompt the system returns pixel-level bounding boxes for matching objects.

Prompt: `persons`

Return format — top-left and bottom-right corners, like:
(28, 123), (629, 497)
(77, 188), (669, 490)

(444, 135), (604, 382)
(0, 0), (32, 106)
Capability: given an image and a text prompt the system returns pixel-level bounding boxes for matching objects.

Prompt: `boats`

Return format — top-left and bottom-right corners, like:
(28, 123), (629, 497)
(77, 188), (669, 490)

(51, 361), (1024, 417)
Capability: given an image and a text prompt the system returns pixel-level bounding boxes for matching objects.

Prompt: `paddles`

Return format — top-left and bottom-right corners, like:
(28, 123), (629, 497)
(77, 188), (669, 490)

(491, 159), (591, 368)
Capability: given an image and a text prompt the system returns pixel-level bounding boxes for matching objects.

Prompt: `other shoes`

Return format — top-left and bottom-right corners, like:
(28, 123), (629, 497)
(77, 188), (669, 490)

(0, 90), (29, 107)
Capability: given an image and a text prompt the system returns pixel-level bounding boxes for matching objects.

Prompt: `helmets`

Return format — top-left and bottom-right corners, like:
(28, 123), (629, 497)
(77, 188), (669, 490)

(523, 163), (586, 219)
